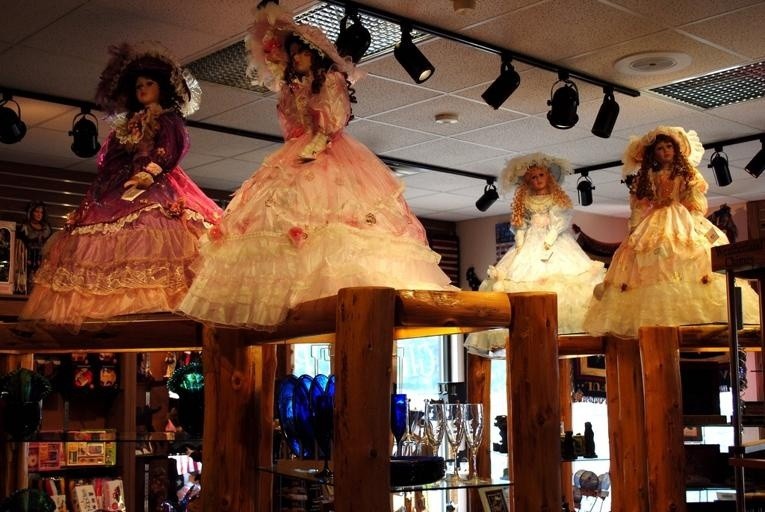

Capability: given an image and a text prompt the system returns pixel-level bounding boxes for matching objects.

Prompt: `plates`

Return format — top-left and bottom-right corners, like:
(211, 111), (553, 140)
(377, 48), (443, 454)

(276, 374), (335, 461)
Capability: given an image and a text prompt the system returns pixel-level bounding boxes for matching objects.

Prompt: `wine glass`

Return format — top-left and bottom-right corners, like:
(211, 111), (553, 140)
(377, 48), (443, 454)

(389, 394), (407, 459)
(425, 403), (489, 485)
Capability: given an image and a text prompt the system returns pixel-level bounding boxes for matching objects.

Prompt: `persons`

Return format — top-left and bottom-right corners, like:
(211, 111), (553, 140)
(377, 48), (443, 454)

(16, 198), (53, 293)
(93, 62), (188, 315)
(632, 136), (711, 327)
(273, 32), (353, 299)
(510, 165), (573, 334)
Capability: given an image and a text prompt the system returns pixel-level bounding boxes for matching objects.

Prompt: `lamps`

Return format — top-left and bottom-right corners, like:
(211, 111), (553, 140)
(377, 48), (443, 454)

(0, 81), (102, 158)
(476, 173), (499, 212)
(571, 132), (765, 205)
(321, 1), (640, 137)
